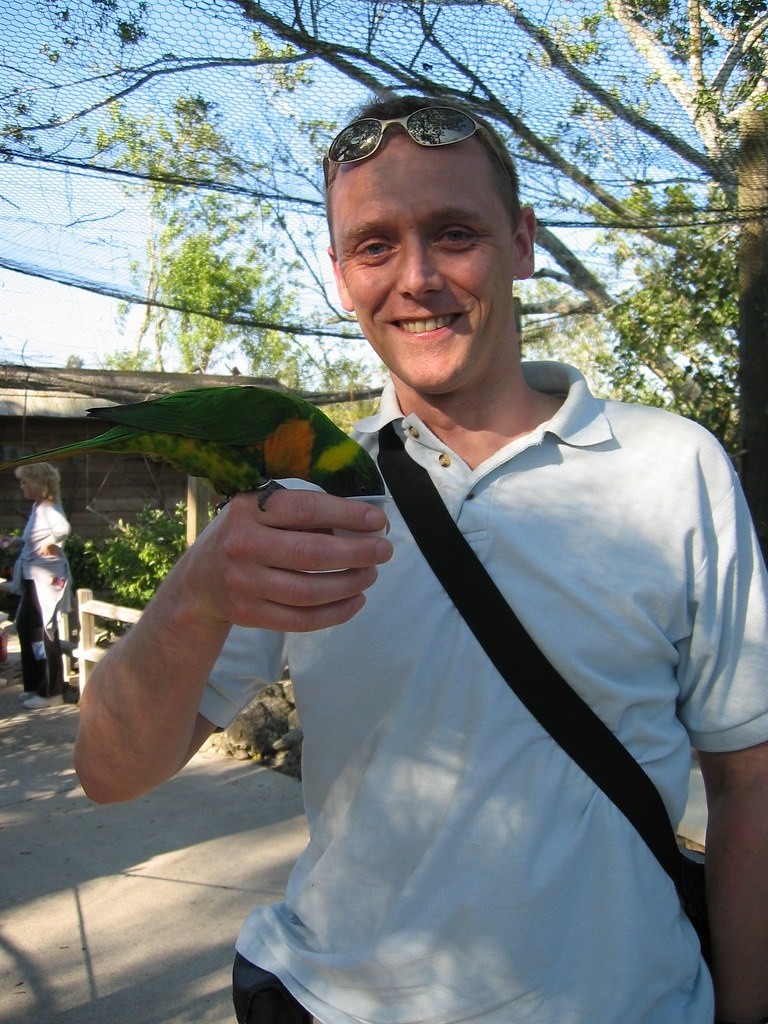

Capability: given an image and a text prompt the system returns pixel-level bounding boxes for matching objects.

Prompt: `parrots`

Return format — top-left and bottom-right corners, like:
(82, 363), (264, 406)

(0, 385), (385, 515)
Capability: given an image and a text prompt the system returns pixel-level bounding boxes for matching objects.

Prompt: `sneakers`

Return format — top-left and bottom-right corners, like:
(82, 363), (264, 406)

(19, 692), (37, 700)
(23, 695), (63, 708)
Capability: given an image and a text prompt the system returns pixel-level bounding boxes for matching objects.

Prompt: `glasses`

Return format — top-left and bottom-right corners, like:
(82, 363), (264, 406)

(323, 106), (514, 194)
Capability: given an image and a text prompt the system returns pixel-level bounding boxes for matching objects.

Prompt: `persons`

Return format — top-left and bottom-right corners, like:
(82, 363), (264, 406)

(0, 462), (71, 708)
(70, 97), (768, 1024)
